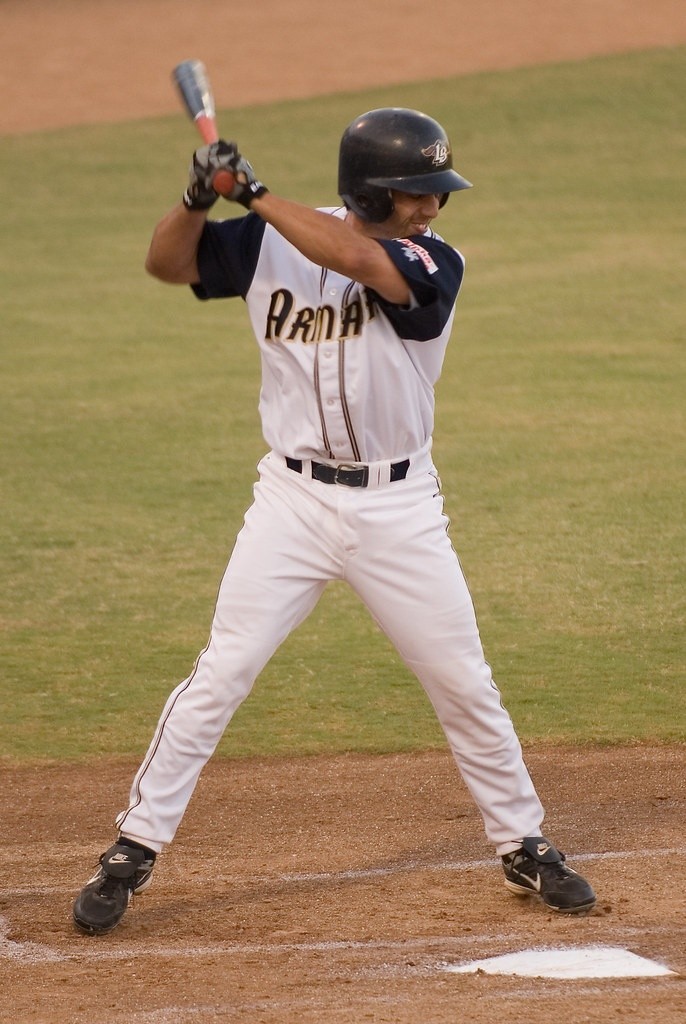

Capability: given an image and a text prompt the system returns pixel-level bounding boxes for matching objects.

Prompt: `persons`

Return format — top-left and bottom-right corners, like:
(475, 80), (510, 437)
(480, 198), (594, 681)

(74, 105), (595, 934)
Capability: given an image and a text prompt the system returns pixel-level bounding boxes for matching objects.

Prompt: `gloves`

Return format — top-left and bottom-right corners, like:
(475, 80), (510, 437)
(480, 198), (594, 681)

(182, 141), (237, 211)
(204, 151), (269, 209)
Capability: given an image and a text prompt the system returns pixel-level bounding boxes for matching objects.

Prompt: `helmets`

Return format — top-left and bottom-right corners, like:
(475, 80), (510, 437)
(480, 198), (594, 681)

(338, 108), (473, 224)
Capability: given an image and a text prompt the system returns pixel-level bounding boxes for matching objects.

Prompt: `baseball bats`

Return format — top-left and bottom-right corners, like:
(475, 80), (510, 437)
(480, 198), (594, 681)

(171, 59), (236, 196)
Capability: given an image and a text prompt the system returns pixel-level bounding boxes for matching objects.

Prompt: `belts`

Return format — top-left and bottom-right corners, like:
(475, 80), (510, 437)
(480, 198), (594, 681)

(286, 456), (409, 488)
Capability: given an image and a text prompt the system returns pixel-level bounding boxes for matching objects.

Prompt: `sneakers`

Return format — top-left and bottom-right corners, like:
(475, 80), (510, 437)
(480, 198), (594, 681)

(73, 837), (155, 936)
(501, 837), (598, 912)
